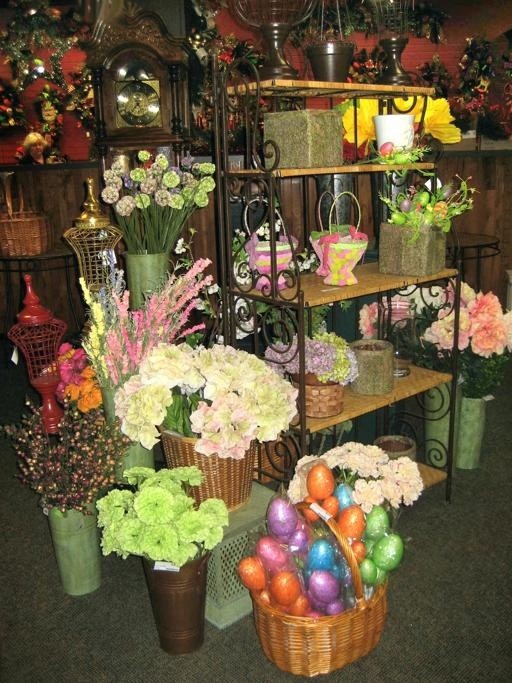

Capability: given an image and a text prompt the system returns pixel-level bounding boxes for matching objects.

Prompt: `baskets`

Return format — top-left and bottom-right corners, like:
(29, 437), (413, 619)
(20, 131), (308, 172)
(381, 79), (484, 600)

(253, 433), (296, 484)
(0, 172), (55, 258)
(318, 191), (368, 287)
(289, 375), (344, 418)
(309, 192), (339, 276)
(244, 199), (298, 292)
(157, 425), (257, 514)
(248, 502), (388, 678)
(374, 434), (416, 462)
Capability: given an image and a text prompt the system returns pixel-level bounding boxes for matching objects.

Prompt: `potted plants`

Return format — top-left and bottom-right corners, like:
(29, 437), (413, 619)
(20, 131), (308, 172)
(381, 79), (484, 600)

(379, 173), (485, 278)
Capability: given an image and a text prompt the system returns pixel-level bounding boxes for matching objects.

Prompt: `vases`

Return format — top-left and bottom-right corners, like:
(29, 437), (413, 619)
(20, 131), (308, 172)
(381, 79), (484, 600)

(424, 380), (462, 469)
(46, 505), (103, 598)
(141, 553), (210, 655)
(100, 386), (155, 486)
(121, 251), (171, 312)
(454, 396), (485, 470)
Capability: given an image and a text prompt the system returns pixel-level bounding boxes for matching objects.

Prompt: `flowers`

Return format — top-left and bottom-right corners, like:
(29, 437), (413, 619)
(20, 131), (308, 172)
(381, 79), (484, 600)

(4, 396), (134, 517)
(423, 289), (510, 398)
(113, 342), (299, 460)
(95, 464), (230, 566)
(54, 343), (103, 414)
(102, 149), (215, 254)
(359, 273), (477, 353)
(78, 246), (213, 389)
(265, 331), (359, 387)
(287, 442), (425, 515)
